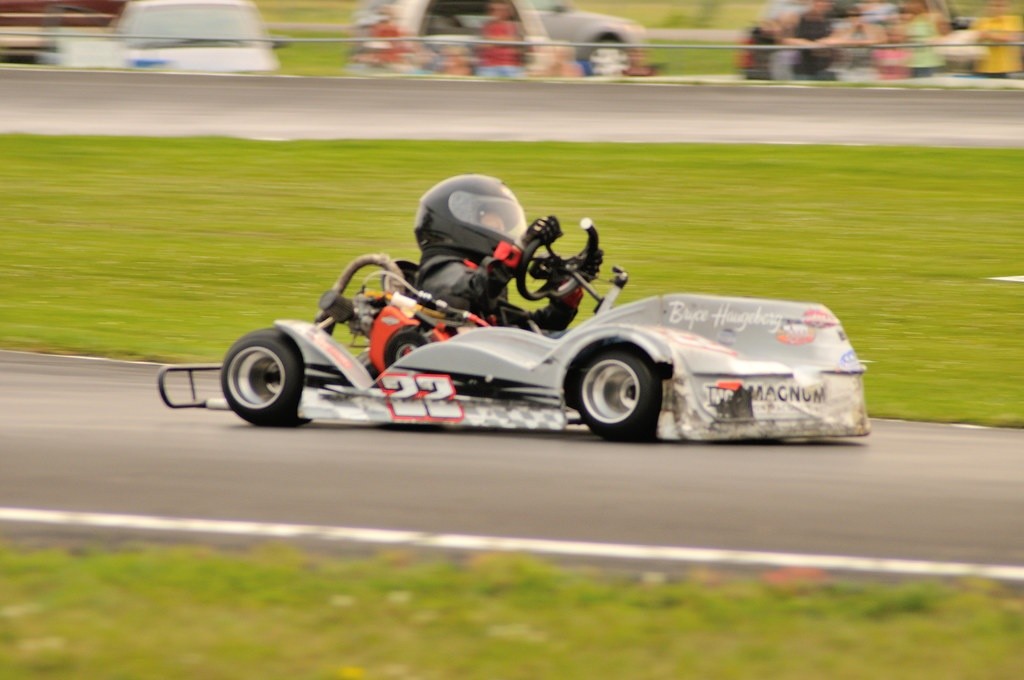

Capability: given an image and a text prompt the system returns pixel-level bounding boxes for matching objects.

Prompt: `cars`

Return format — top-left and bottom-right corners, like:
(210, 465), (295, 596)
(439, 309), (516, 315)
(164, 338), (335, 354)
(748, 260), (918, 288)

(36, 0), (284, 74)
(393, 0), (646, 79)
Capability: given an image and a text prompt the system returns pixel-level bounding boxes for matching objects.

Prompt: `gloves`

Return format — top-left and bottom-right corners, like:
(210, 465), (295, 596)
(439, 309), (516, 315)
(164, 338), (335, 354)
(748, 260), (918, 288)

(591, 249), (604, 273)
(525, 215), (561, 245)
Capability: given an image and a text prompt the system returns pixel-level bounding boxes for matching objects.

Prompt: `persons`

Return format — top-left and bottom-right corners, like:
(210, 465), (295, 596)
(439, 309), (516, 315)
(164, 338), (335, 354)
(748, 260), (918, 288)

(350, 0), (659, 76)
(413, 174), (604, 337)
(730, 0), (1024, 82)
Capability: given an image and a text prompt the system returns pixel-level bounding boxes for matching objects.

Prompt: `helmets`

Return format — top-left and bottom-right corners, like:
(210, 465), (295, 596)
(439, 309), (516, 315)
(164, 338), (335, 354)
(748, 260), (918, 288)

(414, 173), (528, 260)
